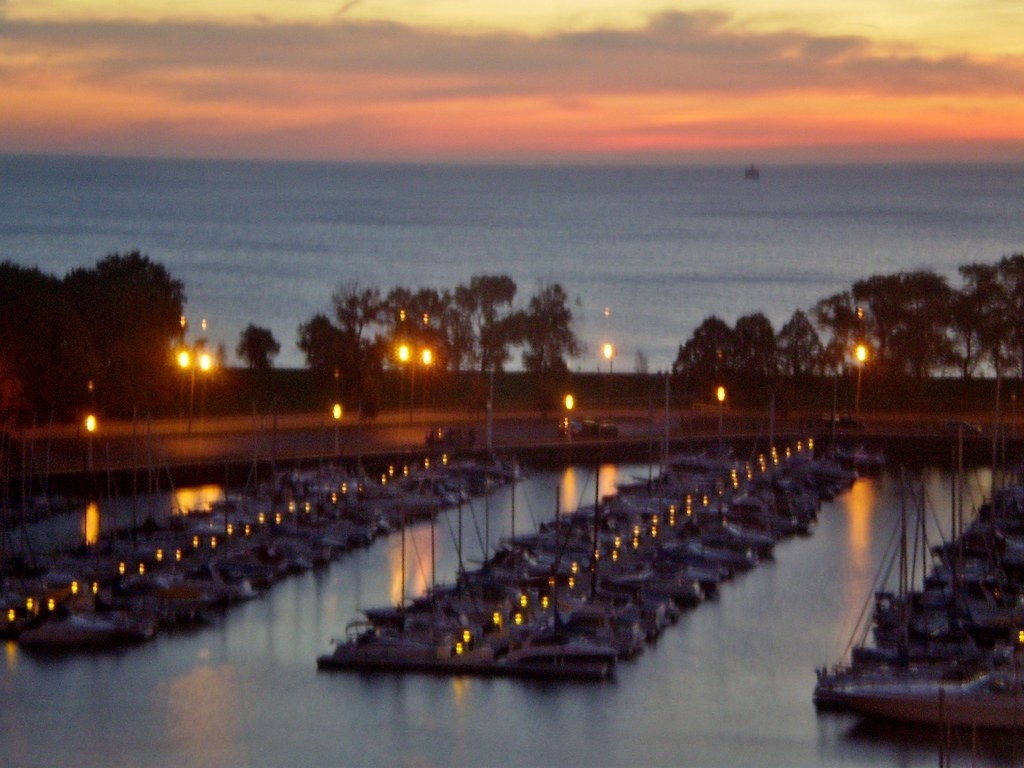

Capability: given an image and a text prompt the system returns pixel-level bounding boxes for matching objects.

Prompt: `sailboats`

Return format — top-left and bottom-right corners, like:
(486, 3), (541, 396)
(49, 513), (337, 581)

(0, 358), (1024, 734)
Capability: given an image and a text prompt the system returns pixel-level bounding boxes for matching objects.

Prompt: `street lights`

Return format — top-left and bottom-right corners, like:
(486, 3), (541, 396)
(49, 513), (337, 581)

(86, 414), (97, 469)
(180, 349), (194, 437)
(421, 347), (433, 424)
(332, 400), (342, 452)
(716, 386), (727, 436)
(854, 346), (868, 431)
(397, 343), (417, 428)
(200, 353), (211, 431)
(602, 343), (615, 419)
(563, 392), (575, 445)
